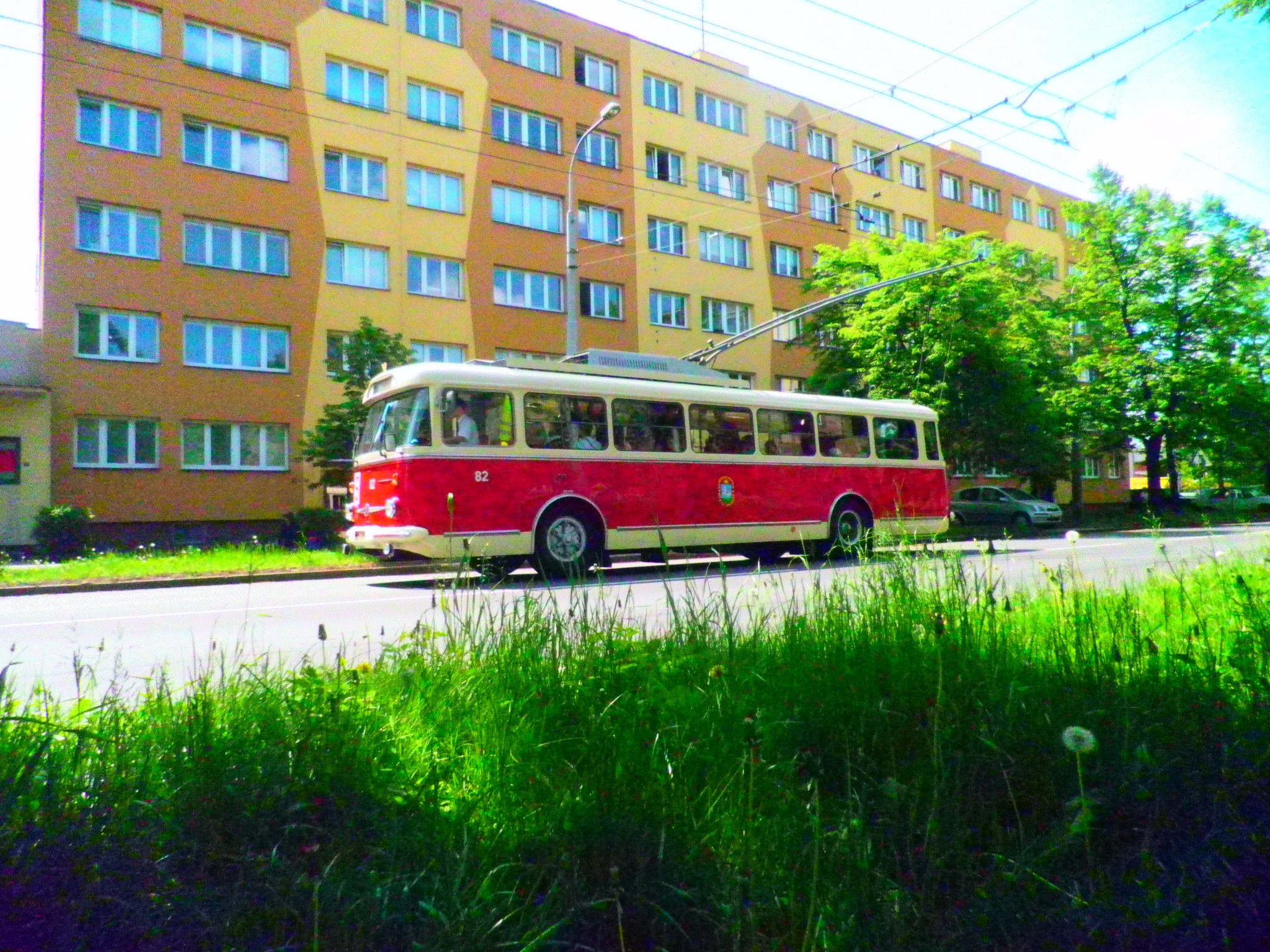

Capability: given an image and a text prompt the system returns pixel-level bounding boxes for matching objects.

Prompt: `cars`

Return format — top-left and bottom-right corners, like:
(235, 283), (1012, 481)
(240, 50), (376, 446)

(949, 485), (1063, 529)
(1210, 488), (1270, 509)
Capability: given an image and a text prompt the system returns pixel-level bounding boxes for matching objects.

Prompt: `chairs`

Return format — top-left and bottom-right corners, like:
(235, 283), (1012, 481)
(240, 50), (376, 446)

(486, 411), (911, 461)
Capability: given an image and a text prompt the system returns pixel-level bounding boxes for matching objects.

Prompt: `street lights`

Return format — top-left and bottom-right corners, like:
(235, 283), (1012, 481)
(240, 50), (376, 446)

(563, 101), (622, 356)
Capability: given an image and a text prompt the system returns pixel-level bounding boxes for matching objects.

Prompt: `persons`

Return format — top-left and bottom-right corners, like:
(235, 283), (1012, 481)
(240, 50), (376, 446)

(418, 397), (481, 446)
(530, 402), (919, 464)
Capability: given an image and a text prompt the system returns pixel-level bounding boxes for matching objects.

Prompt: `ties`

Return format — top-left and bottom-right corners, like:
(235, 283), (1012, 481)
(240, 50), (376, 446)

(456, 419), (459, 437)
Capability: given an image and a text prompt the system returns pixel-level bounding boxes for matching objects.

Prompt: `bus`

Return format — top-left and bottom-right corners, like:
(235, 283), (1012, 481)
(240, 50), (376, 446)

(343, 347), (949, 579)
